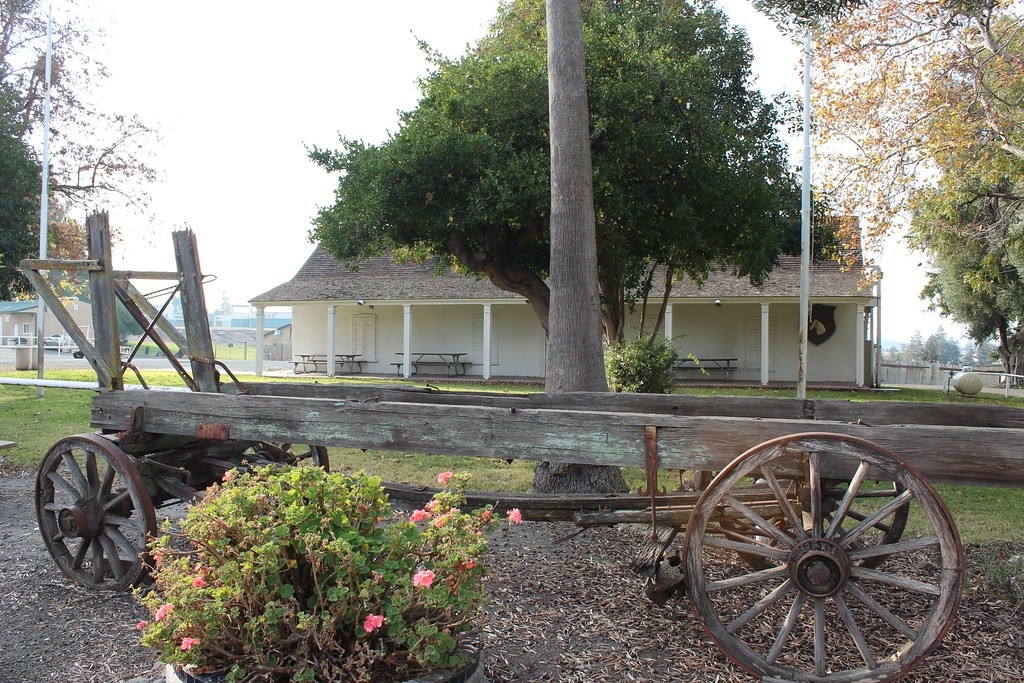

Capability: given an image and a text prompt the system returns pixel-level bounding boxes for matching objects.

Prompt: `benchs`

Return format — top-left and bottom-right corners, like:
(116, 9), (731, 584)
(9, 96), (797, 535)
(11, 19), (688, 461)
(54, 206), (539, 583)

(672, 366), (738, 369)
(289, 361), (348, 364)
(308, 360), (367, 363)
(389, 362), (472, 366)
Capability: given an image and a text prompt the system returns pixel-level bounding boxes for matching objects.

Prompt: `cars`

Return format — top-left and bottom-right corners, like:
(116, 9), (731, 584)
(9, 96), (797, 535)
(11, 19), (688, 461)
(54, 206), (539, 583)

(43, 333), (69, 352)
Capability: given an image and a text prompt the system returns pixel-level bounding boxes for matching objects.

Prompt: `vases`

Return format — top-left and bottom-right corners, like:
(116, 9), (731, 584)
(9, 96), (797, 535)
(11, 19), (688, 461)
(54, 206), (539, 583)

(165, 661), (483, 683)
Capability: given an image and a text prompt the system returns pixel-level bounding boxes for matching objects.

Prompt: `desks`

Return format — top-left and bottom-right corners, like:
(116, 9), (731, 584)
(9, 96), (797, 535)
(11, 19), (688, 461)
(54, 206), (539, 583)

(672, 358), (738, 382)
(395, 352), (467, 377)
(294, 354), (362, 375)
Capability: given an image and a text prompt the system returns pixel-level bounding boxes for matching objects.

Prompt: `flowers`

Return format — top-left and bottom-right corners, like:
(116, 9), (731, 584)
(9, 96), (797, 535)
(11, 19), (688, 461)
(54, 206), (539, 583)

(129, 456), (523, 677)
